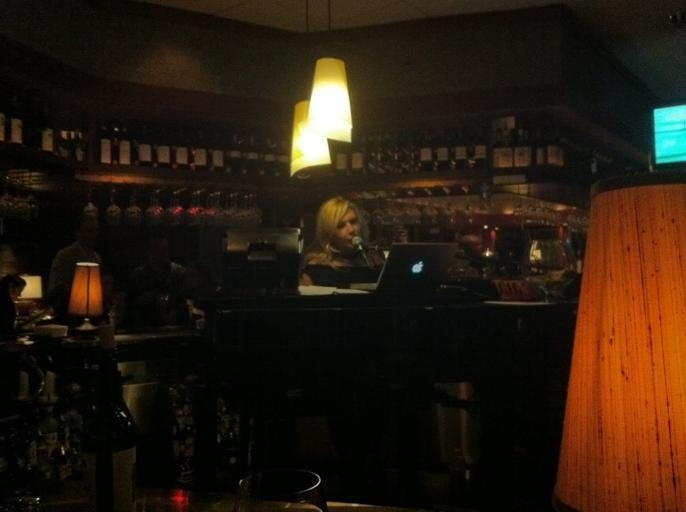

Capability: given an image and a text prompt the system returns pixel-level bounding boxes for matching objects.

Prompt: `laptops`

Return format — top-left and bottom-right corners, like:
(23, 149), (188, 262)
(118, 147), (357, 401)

(350, 241), (458, 293)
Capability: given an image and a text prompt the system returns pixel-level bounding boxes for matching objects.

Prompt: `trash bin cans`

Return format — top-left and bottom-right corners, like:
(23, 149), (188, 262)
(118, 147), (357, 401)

(428, 381), (484, 465)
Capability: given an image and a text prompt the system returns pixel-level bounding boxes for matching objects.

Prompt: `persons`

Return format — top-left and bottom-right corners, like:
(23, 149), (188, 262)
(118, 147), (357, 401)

(298, 197), (383, 285)
(126, 228), (187, 333)
(1, 273), (46, 394)
(48, 218), (117, 311)
(446, 233), (485, 277)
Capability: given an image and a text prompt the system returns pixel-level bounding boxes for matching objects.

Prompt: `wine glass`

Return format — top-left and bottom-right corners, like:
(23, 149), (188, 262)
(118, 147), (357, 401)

(65, 190), (560, 235)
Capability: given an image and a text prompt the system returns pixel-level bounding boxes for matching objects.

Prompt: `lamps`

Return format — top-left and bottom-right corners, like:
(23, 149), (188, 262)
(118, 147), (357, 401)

(309, 0), (355, 146)
(66, 260), (106, 338)
(548, 167), (685, 511)
(288, 1), (335, 179)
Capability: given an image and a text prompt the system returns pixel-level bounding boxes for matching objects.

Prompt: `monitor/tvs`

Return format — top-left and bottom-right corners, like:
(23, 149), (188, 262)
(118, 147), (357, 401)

(645, 100), (686, 168)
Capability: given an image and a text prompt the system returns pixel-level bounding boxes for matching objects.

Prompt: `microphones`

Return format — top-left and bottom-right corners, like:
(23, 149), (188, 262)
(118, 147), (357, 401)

(351, 235), (370, 266)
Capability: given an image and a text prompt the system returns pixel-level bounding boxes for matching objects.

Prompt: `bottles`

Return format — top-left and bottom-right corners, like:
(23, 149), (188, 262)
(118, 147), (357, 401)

(8, 116), (597, 183)
(0, 350), (136, 511)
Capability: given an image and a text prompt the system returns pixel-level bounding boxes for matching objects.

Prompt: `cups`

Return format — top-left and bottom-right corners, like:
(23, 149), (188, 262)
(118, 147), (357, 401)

(236, 468), (328, 512)
